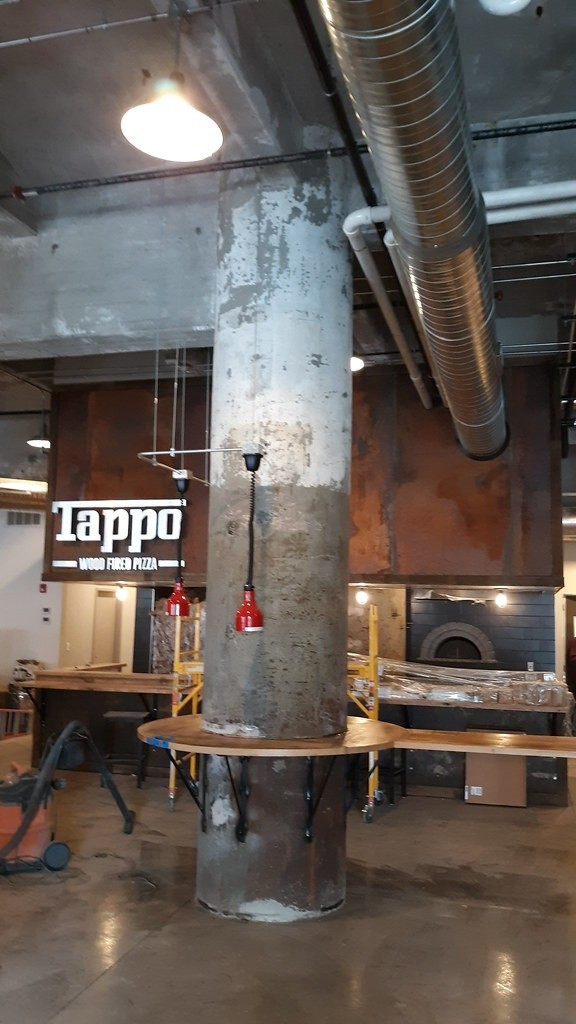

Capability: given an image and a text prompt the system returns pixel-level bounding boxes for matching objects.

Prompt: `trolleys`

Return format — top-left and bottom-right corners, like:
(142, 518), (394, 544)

(168, 604), (384, 824)
(0, 731), (90, 876)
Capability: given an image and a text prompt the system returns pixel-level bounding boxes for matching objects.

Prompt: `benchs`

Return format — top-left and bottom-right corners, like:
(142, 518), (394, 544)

(386, 727), (576, 806)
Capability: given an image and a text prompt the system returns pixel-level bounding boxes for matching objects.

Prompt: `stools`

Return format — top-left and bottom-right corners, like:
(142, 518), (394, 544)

(101, 712), (150, 788)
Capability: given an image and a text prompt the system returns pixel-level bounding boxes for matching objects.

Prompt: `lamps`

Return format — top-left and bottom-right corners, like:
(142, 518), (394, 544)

(165, 477), (193, 616)
(235, 453), (267, 631)
(116, 0), (224, 161)
(26, 399), (51, 449)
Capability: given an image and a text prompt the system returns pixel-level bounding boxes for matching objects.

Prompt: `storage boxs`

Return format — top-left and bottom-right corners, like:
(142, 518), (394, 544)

(463, 729), (529, 808)
